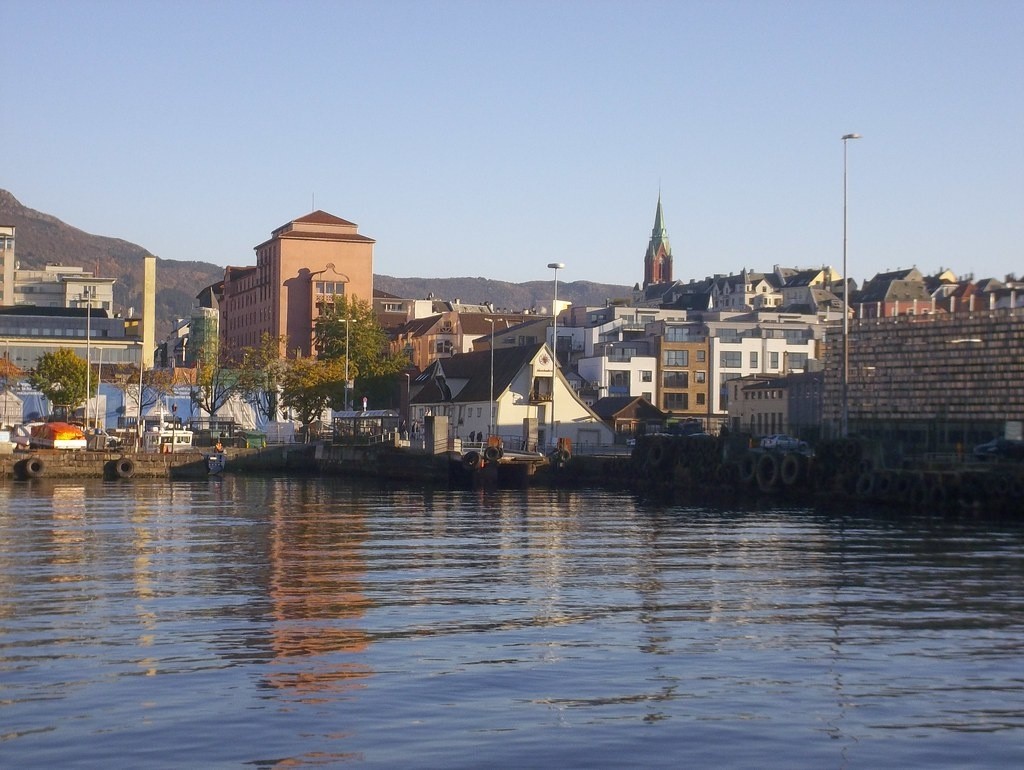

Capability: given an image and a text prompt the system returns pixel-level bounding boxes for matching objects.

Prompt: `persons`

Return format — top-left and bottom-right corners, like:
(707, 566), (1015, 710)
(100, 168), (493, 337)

(412, 421), (419, 441)
(469, 430), (475, 445)
(477, 431), (482, 445)
(400, 419), (406, 440)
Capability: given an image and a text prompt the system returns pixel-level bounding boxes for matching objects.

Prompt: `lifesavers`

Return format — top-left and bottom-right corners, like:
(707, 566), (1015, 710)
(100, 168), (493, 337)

(459, 444), (505, 475)
(114, 457), (136, 480)
(561, 448), (572, 463)
(24, 456), (47, 479)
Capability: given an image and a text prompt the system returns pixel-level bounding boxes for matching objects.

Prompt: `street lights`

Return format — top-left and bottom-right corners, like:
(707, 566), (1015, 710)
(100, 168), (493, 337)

(547, 262), (566, 446)
(93, 346), (102, 428)
(405, 374), (410, 430)
(721, 359), (728, 427)
(83, 290), (91, 430)
(134, 341), (144, 428)
(171, 401), (177, 454)
(483, 317), (494, 438)
(841, 133), (862, 441)
(337, 314), (357, 411)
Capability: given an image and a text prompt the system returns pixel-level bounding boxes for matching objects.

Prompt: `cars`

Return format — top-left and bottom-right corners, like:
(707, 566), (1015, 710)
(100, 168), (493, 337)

(11, 420), (244, 453)
(760, 433), (809, 453)
